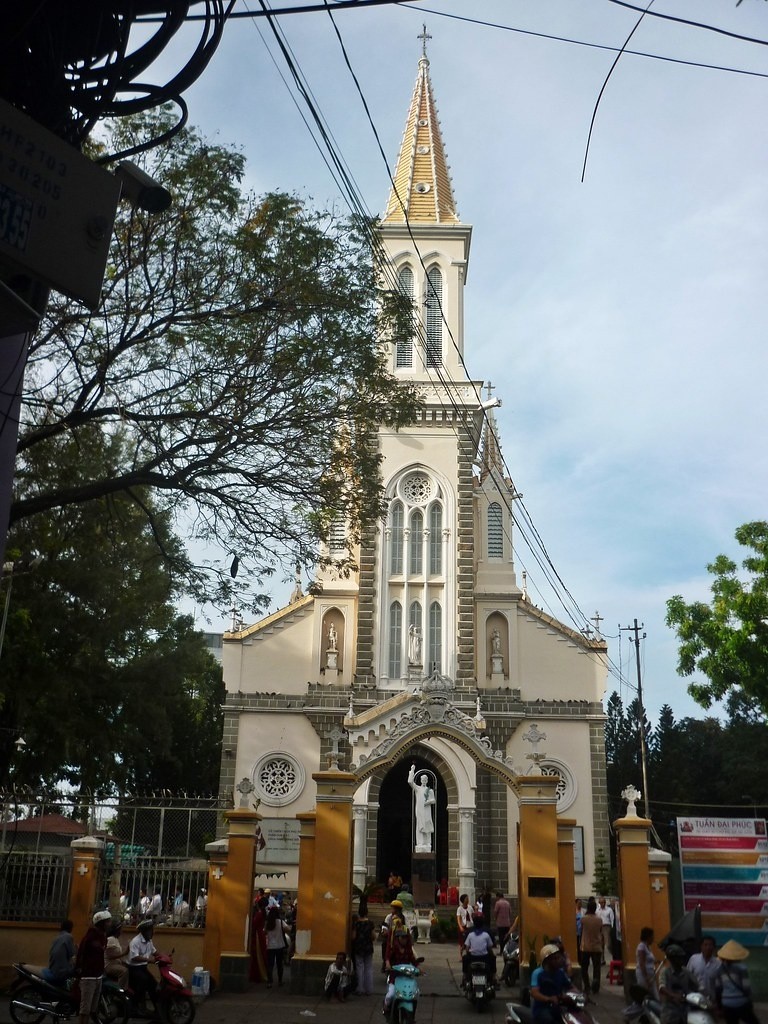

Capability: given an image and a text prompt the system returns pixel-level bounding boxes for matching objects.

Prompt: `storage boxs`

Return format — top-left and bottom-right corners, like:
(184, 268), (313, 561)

(193, 971), (209, 994)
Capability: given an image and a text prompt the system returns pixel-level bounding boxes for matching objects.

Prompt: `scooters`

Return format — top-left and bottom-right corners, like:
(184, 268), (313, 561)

(381, 956), (426, 1024)
(505, 991), (599, 1024)
(634, 991), (716, 1024)
(128, 946), (196, 1024)
(501, 933), (520, 986)
(459, 951), (496, 1011)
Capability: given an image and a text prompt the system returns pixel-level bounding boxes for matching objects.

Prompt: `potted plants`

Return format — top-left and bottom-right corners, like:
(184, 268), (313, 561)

(437, 917), (455, 943)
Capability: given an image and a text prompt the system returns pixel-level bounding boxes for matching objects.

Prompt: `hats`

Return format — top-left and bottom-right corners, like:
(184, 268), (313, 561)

(666, 944), (685, 957)
(92, 910), (111, 926)
(264, 888), (271, 893)
(717, 939), (750, 960)
(136, 919), (152, 931)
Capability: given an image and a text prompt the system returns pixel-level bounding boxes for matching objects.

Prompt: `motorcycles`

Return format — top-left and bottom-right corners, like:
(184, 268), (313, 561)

(7, 956), (131, 1024)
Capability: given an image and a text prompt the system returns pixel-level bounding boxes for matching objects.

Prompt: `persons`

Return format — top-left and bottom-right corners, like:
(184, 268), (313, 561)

(322, 619), (338, 649)
(408, 762), (437, 853)
(407, 624), (423, 665)
(49, 870), (753, 1023)
(490, 627), (501, 654)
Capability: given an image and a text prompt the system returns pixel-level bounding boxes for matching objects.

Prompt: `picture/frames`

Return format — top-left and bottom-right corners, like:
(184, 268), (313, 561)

(255, 817), (300, 865)
(570, 826), (585, 873)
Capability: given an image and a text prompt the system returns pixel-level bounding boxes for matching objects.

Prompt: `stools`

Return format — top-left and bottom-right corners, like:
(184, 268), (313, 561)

(610, 961), (622, 984)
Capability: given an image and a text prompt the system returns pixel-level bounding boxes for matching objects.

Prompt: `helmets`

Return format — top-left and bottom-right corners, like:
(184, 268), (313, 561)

(540, 944), (559, 964)
(394, 930), (410, 937)
(390, 900), (403, 908)
